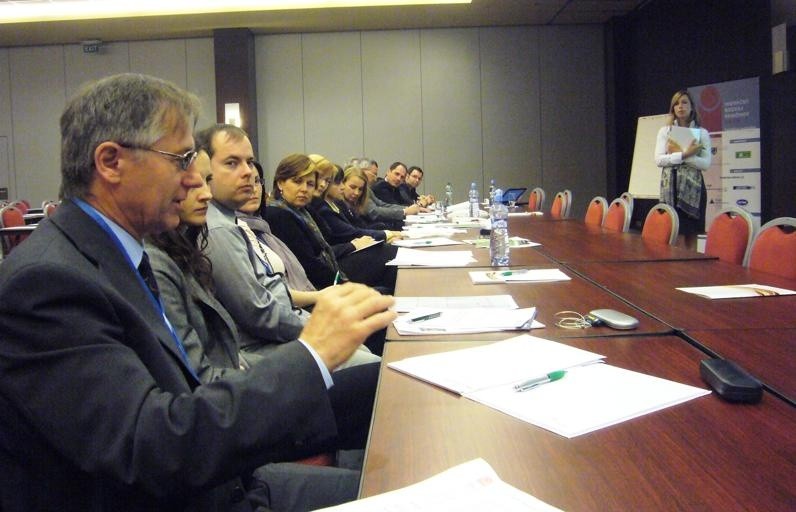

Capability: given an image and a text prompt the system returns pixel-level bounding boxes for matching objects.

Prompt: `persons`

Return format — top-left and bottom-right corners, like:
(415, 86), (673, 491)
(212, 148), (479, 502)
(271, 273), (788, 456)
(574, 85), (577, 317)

(0, 73), (396, 512)
(654, 90), (711, 251)
(139, 125), (381, 447)
(141, 125), (434, 384)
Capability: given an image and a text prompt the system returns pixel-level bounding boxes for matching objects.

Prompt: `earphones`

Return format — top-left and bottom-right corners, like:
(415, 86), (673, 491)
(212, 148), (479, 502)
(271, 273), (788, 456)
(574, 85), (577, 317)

(585, 315), (601, 325)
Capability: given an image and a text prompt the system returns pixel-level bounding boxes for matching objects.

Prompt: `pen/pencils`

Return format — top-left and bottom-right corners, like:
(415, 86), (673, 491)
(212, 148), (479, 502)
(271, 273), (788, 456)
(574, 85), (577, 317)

(501, 269), (527, 275)
(515, 370), (568, 393)
(412, 312), (442, 322)
(333, 271), (340, 286)
(412, 241), (433, 245)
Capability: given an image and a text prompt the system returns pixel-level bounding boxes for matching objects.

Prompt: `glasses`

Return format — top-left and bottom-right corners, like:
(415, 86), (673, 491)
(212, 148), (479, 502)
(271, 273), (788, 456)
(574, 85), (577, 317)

(253, 178), (266, 186)
(121, 143), (198, 170)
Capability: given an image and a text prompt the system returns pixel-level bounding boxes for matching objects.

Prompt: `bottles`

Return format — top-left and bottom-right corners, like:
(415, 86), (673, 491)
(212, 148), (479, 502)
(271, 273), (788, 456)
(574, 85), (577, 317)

(445, 183), (451, 206)
(469, 180), (509, 266)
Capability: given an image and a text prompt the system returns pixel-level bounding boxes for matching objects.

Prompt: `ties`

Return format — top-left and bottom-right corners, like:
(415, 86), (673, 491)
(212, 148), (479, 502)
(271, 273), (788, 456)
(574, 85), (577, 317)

(137, 248), (162, 313)
(235, 216), (266, 263)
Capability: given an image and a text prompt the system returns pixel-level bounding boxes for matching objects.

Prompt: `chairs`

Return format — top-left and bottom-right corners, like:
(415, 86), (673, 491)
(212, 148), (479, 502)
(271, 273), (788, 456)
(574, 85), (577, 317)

(528, 188), (796, 284)
(0, 198), (62, 259)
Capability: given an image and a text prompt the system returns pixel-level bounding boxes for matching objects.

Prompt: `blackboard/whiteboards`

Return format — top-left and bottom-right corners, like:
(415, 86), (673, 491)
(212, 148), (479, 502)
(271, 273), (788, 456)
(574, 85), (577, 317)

(628, 114), (672, 199)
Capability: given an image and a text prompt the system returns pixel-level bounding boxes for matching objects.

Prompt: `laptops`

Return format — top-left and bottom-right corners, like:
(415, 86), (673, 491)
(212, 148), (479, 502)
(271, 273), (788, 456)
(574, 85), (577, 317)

(495, 188), (529, 206)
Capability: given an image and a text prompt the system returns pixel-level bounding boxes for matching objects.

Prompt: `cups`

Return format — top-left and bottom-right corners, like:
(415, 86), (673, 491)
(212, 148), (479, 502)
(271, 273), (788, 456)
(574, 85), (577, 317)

(435, 201), (442, 218)
(509, 193), (517, 208)
